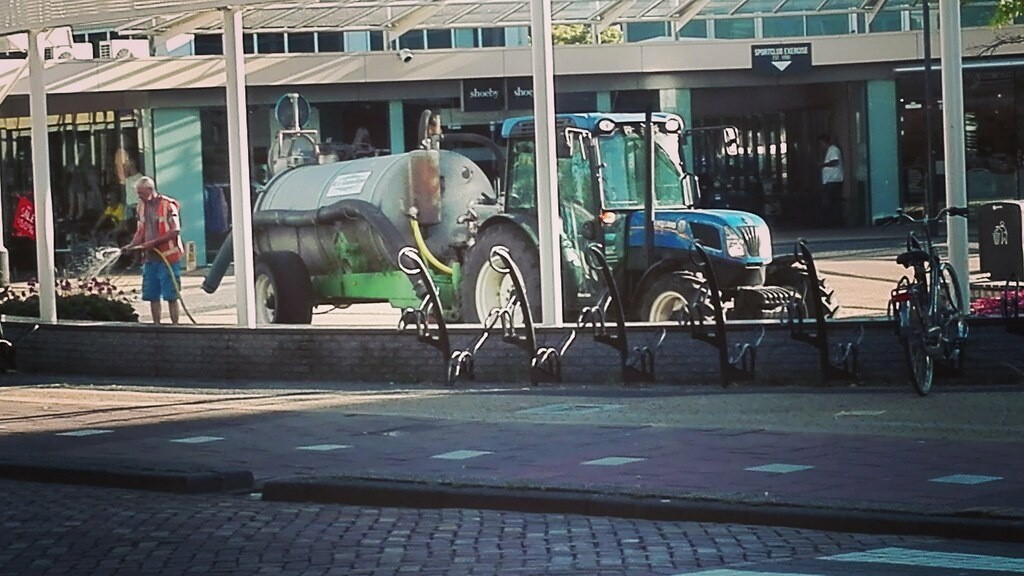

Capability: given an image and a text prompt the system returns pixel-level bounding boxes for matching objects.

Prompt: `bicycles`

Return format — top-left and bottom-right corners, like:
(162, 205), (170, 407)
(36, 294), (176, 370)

(869, 205), (973, 397)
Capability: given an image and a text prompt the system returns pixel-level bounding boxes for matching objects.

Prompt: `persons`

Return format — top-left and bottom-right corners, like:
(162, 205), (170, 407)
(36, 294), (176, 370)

(816, 134), (844, 223)
(91, 160), (145, 270)
(121, 176), (185, 325)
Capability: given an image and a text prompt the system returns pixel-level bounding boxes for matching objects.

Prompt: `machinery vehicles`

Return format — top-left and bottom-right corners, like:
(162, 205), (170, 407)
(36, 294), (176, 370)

(203, 89), (777, 323)
